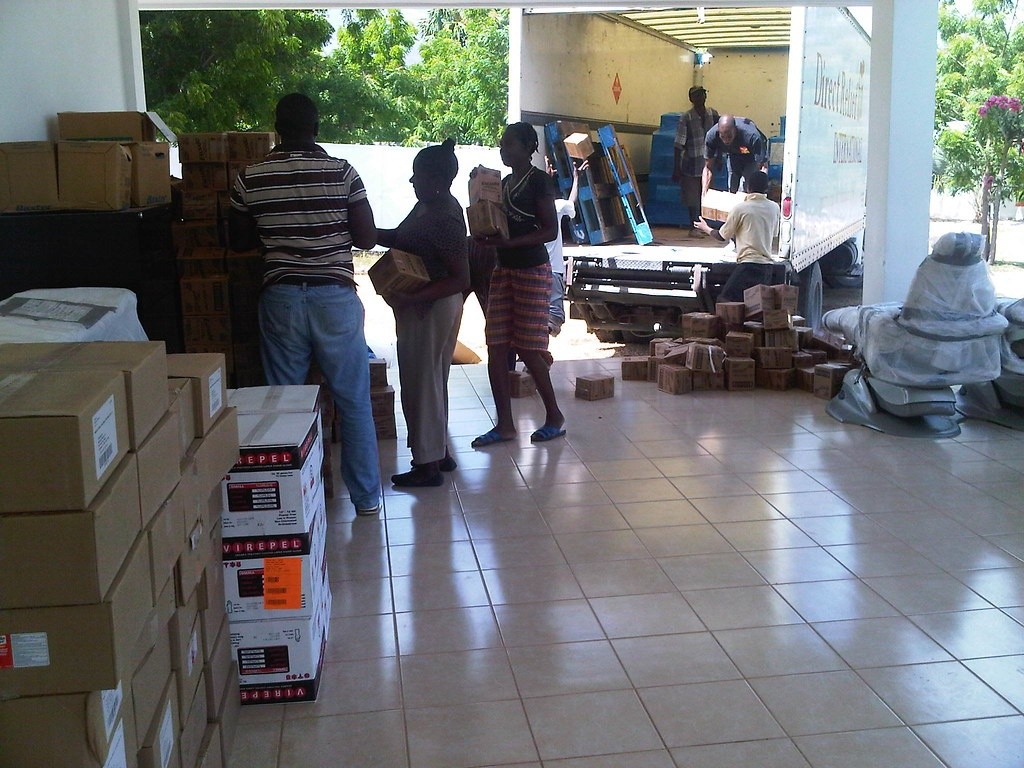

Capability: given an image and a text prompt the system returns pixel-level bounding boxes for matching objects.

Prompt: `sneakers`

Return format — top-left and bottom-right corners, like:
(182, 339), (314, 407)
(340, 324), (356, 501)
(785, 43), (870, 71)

(357, 495), (382, 515)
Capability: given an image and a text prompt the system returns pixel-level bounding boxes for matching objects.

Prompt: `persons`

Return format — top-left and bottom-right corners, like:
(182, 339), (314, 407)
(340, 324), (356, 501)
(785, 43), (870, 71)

(693, 171), (780, 303)
(374, 137), (469, 487)
(672, 86), (719, 238)
(543, 155), (589, 337)
(225, 92), (375, 516)
(469, 121), (567, 446)
(701, 114), (767, 198)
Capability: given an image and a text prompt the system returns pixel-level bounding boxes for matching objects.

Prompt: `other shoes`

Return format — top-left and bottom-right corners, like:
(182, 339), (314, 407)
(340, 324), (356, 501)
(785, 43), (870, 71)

(391, 468), (444, 486)
(688, 229), (705, 238)
(410, 457), (457, 472)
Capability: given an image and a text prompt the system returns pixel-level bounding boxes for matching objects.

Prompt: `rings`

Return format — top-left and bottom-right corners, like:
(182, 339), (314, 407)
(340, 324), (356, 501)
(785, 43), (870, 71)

(485, 236), (489, 241)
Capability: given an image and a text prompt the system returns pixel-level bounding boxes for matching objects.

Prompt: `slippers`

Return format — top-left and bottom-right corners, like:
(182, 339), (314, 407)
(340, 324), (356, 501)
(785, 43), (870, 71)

(531, 426), (566, 442)
(471, 431), (515, 447)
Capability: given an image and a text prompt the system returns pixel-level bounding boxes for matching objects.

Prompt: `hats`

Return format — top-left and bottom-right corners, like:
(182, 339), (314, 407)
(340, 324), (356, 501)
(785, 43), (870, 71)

(689, 86), (706, 97)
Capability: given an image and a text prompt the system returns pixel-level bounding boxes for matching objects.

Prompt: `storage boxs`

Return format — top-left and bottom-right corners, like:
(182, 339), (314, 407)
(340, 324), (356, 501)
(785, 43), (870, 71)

(507, 370), (536, 398)
(466, 199), (509, 239)
(563, 132), (594, 161)
(368, 247), (431, 297)
(620, 284), (857, 400)
(0, 112), (394, 768)
(574, 373), (614, 401)
(702, 189), (749, 222)
(468, 166), (503, 206)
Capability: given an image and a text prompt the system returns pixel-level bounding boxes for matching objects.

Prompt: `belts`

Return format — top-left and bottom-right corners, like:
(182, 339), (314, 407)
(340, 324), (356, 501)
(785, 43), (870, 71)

(266, 276), (344, 286)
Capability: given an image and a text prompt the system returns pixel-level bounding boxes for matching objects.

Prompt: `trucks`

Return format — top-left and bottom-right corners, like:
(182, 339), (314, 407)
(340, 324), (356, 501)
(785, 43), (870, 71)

(508, 7), (870, 328)
